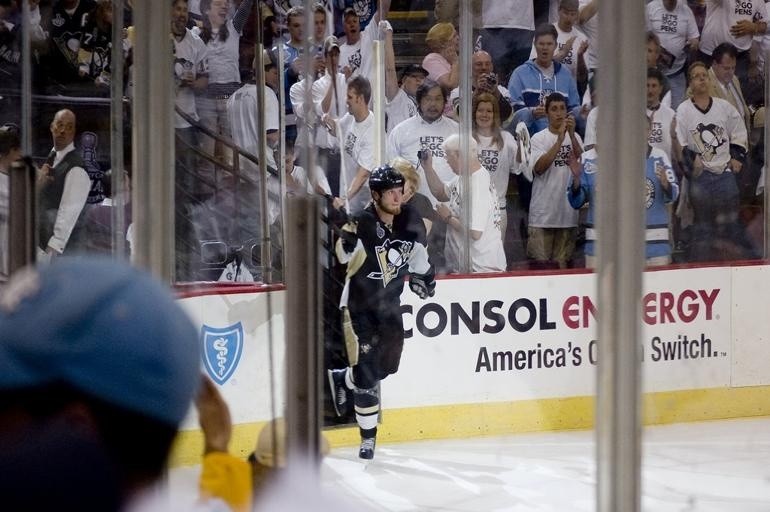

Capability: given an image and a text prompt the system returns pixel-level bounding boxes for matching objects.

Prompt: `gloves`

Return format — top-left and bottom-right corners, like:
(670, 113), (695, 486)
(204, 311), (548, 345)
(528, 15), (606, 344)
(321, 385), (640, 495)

(408, 265), (437, 299)
(340, 220), (358, 254)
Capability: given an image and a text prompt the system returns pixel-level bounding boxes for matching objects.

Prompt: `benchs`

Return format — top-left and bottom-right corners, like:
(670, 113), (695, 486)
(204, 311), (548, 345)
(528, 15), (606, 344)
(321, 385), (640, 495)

(385, 0), (434, 79)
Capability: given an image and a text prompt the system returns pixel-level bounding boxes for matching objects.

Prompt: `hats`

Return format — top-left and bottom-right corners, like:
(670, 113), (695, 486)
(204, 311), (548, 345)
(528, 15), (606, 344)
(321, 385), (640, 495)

(255, 417), (329, 469)
(0, 253), (198, 429)
(253, 48), (289, 69)
(324, 35), (341, 53)
(341, 6), (359, 24)
(403, 63), (430, 77)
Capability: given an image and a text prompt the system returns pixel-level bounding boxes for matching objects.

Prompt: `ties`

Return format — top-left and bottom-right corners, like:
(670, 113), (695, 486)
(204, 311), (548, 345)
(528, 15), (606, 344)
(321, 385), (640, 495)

(43, 149), (58, 167)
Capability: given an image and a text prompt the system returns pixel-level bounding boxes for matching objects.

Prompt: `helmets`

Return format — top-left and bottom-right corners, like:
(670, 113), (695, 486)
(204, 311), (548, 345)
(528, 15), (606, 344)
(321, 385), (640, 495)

(368, 163), (405, 192)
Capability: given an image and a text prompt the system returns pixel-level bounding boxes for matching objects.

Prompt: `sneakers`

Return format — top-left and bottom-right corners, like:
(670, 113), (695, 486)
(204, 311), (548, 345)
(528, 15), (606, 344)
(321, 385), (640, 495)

(358, 435), (376, 460)
(331, 366), (350, 417)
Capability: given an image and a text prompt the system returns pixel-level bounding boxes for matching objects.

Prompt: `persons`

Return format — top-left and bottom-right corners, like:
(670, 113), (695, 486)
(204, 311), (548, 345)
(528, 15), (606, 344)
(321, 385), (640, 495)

(247, 418), (330, 511)
(0, 254), (231, 512)
(191, 373), (253, 512)
(326, 163), (437, 461)
